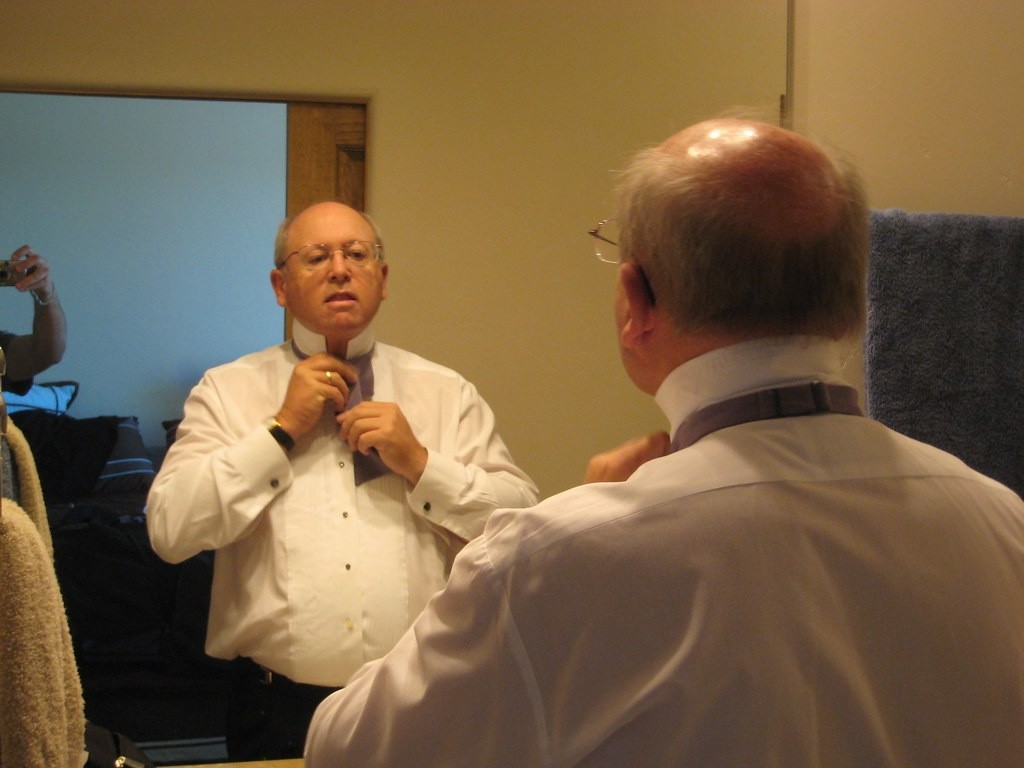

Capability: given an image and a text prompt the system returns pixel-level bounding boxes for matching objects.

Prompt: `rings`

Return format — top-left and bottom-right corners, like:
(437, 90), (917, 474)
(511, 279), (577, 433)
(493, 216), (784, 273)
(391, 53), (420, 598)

(327, 371), (331, 382)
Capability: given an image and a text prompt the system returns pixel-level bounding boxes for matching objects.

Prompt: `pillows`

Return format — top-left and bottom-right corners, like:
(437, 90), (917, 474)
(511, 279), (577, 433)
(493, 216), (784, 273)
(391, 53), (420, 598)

(8, 409), (129, 521)
(65, 413), (159, 523)
(1, 381), (78, 415)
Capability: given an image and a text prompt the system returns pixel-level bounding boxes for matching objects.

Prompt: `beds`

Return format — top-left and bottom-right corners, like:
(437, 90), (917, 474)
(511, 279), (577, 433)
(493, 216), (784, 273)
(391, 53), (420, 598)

(3, 380), (235, 744)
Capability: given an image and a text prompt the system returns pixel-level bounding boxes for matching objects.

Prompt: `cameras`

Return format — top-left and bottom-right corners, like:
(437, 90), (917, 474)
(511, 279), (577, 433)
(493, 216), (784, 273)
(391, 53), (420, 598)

(0, 259), (26, 287)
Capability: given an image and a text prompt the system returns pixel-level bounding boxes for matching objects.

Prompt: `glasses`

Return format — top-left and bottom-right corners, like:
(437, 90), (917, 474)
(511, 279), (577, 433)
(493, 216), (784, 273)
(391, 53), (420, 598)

(588, 218), (657, 309)
(278, 240), (380, 270)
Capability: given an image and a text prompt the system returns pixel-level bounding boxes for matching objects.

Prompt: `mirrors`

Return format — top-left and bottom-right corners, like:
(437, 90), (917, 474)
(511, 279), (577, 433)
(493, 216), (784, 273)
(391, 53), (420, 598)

(0, 0), (794, 768)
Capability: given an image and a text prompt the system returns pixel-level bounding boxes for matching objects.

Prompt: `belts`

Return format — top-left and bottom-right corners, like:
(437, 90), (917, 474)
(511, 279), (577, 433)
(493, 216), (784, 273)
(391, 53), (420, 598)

(247, 666), (287, 697)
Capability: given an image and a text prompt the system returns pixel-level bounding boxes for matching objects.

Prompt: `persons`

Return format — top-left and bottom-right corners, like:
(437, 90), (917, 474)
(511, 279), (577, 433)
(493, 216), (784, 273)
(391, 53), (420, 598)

(302, 116), (1024, 768)
(146, 201), (538, 759)
(0, 245), (67, 438)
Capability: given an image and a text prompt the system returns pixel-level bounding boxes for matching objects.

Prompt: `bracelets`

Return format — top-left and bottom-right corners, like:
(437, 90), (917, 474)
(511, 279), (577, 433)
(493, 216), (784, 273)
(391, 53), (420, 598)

(263, 417), (296, 451)
(32, 285), (56, 305)
